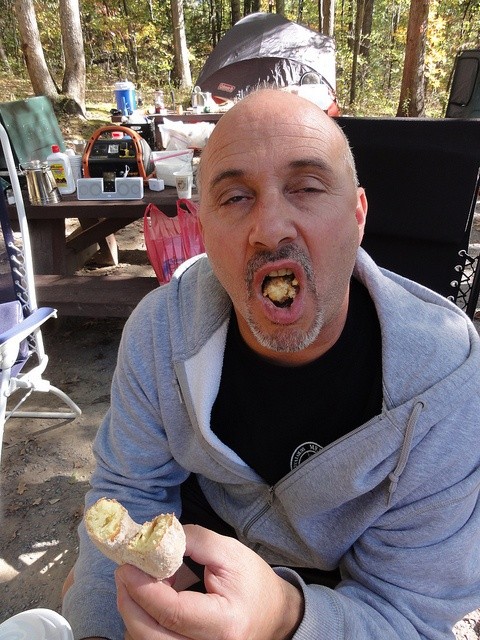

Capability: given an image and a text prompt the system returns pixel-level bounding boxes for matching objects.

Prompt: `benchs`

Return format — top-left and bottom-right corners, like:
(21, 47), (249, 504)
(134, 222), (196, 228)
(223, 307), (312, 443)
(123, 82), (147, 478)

(1, 275), (160, 318)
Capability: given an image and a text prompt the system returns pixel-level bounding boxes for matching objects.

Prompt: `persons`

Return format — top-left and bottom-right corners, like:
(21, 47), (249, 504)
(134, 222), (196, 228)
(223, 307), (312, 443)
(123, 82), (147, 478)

(59, 86), (479, 640)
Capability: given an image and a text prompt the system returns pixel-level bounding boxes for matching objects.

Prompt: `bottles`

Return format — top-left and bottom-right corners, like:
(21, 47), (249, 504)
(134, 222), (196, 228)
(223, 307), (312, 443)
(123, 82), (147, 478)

(47, 145), (76, 194)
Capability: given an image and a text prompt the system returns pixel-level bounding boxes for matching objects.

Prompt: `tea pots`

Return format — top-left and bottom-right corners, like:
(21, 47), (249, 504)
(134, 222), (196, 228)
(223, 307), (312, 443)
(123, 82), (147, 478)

(19, 160), (63, 204)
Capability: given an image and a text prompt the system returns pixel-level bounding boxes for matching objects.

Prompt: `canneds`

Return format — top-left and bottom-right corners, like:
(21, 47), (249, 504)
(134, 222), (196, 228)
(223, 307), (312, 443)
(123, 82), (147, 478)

(176, 102), (183, 117)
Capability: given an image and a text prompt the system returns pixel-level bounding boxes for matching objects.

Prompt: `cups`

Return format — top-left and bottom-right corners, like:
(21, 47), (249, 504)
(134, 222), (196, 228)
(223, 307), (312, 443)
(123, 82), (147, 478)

(172, 171), (193, 199)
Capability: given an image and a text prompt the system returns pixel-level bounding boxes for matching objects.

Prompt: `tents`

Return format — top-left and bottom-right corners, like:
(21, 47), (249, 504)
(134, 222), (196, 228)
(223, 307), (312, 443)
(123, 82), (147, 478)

(444, 50), (480, 118)
(191, 11), (336, 98)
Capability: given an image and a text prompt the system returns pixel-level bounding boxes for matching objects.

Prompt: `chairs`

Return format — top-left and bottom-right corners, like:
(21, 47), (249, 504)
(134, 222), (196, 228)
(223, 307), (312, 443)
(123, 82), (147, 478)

(1, 124), (82, 467)
(335, 116), (480, 325)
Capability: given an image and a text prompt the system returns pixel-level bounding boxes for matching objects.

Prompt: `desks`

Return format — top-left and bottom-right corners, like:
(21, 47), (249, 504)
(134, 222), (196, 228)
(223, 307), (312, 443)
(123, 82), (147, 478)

(148, 112), (225, 148)
(6, 185), (199, 320)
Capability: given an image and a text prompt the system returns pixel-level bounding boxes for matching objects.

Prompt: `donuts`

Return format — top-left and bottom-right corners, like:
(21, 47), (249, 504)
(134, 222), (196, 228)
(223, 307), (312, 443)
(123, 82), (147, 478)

(264, 277), (298, 303)
(81, 498), (188, 582)
